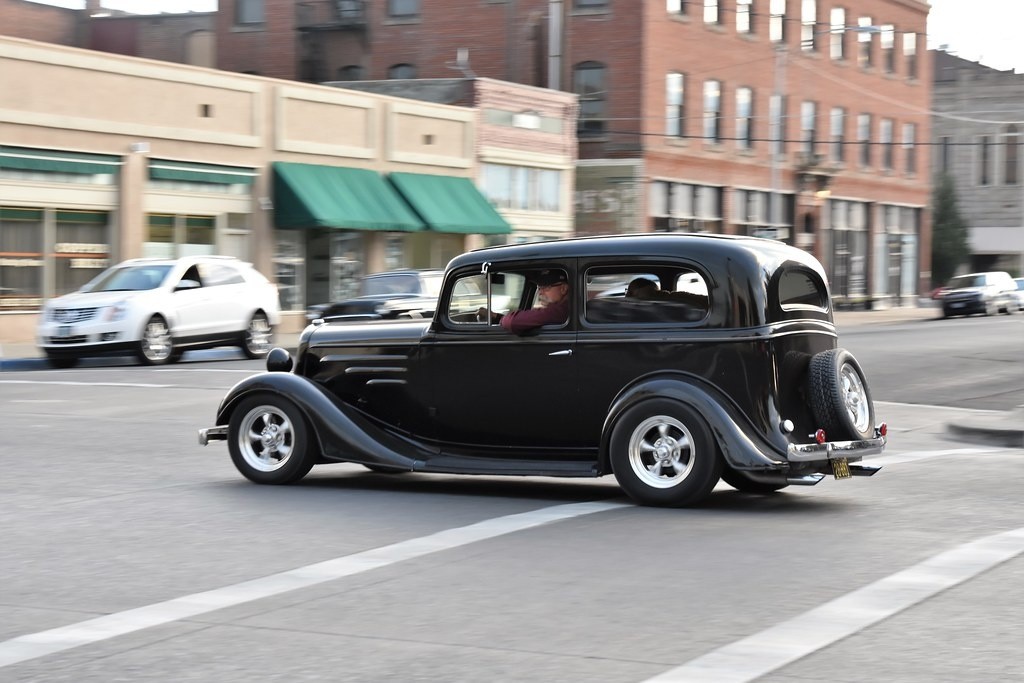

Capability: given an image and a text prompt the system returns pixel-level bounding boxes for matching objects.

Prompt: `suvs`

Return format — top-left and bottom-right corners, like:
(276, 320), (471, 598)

(36, 255), (281, 370)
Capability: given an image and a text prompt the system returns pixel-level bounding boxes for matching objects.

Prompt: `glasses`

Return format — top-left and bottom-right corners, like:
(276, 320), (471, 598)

(625, 285), (641, 294)
(537, 283), (565, 289)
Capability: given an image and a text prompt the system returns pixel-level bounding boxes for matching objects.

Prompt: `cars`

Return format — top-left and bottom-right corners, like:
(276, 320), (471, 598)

(938, 269), (1018, 318)
(196, 231), (886, 509)
(305, 266), (511, 330)
(595, 272), (710, 300)
(1014, 277), (1024, 311)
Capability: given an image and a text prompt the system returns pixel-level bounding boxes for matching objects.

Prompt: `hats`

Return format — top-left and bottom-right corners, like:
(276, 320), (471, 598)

(537, 269), (567, 285)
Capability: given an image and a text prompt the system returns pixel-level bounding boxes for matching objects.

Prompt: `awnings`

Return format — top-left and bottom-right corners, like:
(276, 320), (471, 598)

(273, 161), (512, 238)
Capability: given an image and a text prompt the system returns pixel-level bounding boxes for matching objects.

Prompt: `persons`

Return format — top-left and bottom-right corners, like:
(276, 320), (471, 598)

(475, 267), (571, 333)
(622, 277), (660, 300)
